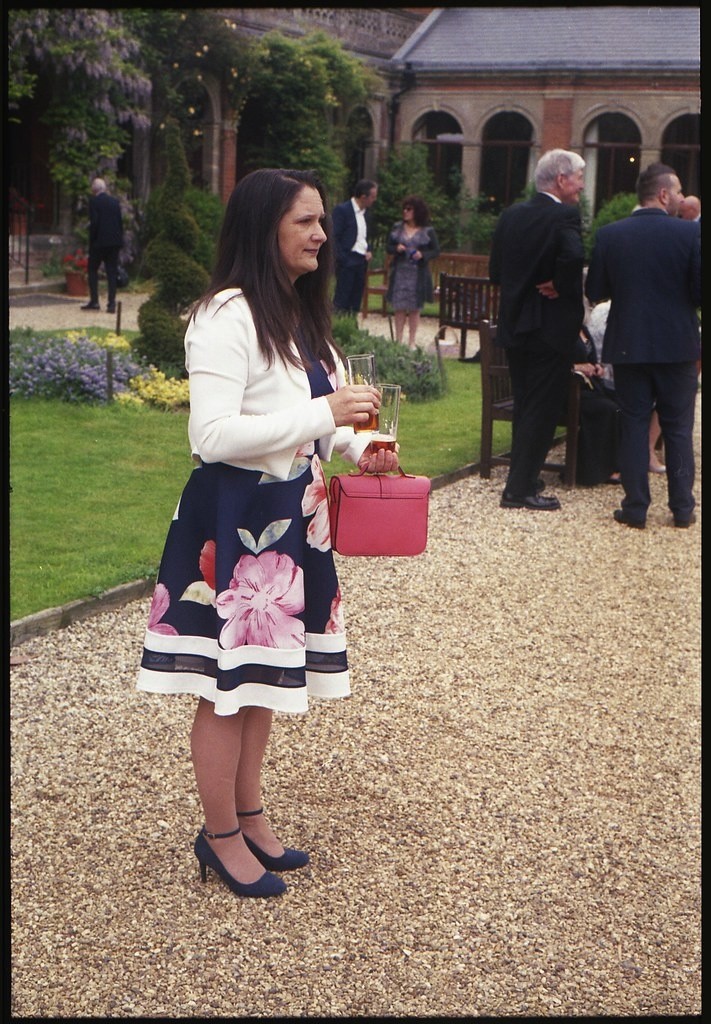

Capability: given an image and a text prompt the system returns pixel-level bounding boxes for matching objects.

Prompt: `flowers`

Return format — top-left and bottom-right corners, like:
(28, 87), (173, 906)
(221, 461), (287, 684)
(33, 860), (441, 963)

(63, 249), (88, 274)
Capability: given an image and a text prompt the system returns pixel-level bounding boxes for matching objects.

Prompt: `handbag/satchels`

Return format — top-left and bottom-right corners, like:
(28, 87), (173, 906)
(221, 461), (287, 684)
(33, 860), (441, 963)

(330, 458), (431, 555)
(427, 326), (462, 359)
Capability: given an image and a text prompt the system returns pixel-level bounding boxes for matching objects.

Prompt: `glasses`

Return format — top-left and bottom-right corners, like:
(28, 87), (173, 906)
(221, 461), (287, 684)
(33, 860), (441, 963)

(401, 206), (415, 212)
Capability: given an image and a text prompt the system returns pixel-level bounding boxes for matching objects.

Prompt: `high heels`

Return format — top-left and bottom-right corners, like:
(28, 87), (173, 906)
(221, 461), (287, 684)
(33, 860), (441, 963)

(235, 809), (309, 870)
(193, 824), (287, 899)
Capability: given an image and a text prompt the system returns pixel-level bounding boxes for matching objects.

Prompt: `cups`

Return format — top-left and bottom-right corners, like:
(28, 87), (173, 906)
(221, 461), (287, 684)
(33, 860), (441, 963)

(370, 384), (401, 459)
(346, 354), (379, 435)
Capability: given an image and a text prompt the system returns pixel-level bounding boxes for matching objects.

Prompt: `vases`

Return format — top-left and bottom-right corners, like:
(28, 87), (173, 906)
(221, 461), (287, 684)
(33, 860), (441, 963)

(66, 273), (89, 296)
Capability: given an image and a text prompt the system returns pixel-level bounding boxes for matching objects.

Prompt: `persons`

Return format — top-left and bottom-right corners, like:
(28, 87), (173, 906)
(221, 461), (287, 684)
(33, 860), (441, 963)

(585, 163), (701, 528)
(81, 179), (124, 313)
(386, 198), (440, 350)
(136, 171), (400, 897)
(332, 180), (378, 326)
(490, 149), (584, 510)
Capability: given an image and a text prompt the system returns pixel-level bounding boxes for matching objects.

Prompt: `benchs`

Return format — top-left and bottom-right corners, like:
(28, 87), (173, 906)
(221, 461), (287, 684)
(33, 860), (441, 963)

(362, 253), (493, 318)
(480, 319), (582, 489)
(438, 271), (500, 358)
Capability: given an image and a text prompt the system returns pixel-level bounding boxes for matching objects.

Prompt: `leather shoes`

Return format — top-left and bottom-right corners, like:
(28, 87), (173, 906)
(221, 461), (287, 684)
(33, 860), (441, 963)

(614, 510), (643, 528)
(536, 480), (545, 493)
(676, 513), (696, 528)
(501, 488), (561, 510)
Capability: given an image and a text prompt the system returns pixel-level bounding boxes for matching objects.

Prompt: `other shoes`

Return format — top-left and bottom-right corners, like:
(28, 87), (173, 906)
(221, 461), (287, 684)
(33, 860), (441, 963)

(604, 472), (622, 485)
(648, 462), (667, 472)
(107, 302), (115, 313)
(81, 301), (100, 310)
